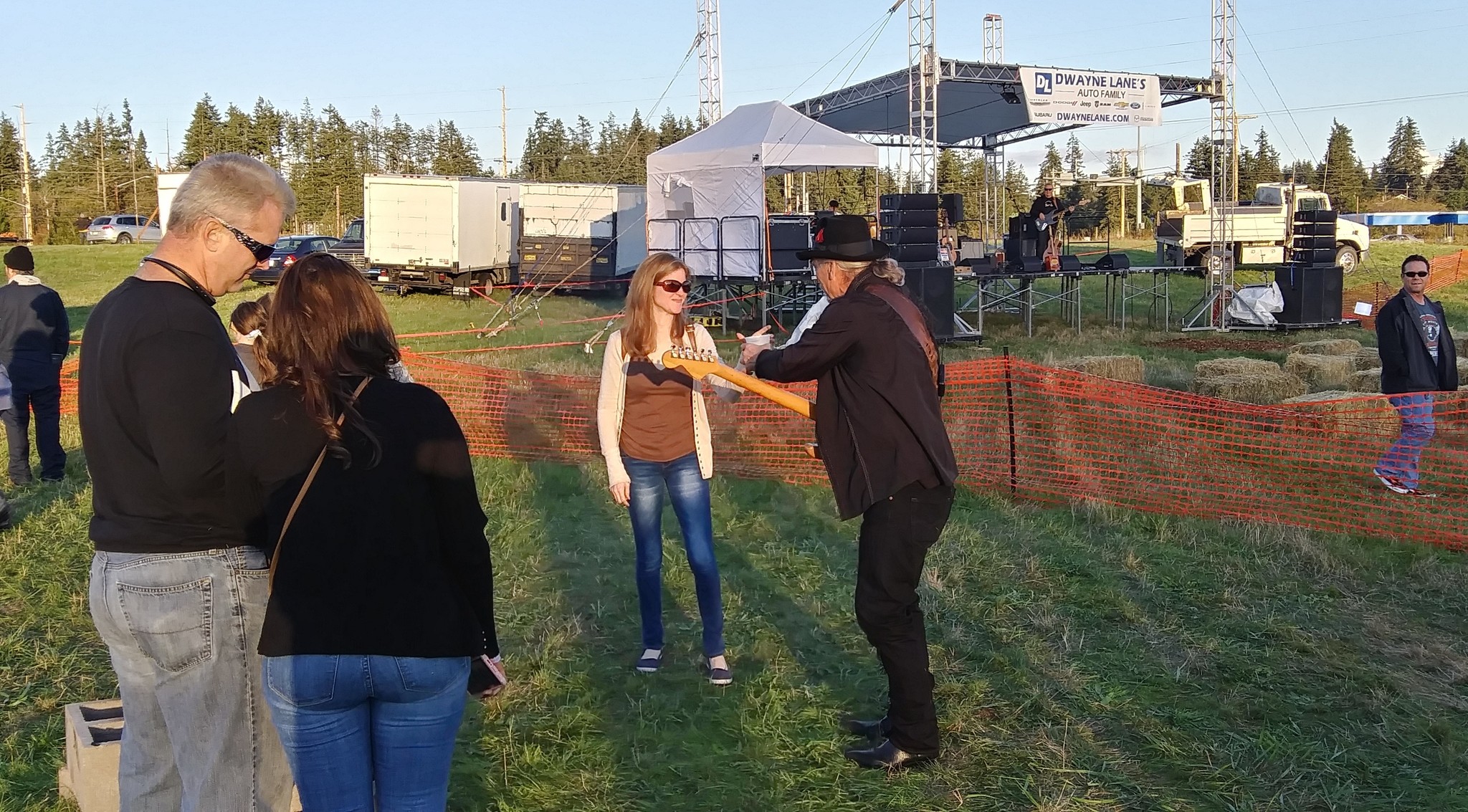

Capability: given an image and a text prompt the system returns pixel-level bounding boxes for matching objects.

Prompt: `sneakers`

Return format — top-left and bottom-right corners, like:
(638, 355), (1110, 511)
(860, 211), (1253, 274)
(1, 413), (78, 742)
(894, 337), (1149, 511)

(1374, 466), (1409, 494)
(1405, 487), (1437, 497)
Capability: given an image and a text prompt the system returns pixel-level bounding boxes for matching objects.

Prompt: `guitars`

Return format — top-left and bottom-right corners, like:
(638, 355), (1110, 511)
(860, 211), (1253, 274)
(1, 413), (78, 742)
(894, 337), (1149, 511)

(1043, 211), (1065, 262)
(940, 209), (957, 262)
(662, 345), (822, 460)
(1035, 198), (1091, 231)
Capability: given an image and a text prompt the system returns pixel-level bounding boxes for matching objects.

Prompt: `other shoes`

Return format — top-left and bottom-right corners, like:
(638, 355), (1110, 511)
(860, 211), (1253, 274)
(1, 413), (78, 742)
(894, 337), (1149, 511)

(636, 650), (663, 673)
(707, 658), (734, 684)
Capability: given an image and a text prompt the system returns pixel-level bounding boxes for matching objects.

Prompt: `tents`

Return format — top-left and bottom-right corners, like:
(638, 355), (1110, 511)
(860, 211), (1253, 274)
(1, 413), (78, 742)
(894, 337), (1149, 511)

(645, 101), (882, 285)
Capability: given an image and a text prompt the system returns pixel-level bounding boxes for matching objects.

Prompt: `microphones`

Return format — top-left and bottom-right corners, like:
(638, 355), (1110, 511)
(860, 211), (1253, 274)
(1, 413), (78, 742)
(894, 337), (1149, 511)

(1005, 187), (1012, 191)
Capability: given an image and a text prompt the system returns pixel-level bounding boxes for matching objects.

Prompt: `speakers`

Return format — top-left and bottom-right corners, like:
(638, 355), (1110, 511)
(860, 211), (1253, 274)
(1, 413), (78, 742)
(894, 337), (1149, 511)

(1274, 210), (1344, 324)
(1095, 253), (1129, 271)
(1004, 216), (1041, 273)
(1057, 255), (1081, 271)
(880, 193), (992, 338)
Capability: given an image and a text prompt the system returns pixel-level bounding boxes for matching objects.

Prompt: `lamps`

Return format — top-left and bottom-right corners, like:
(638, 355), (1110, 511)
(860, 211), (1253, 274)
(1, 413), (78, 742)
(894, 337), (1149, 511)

(1000, 82), (1021, 104)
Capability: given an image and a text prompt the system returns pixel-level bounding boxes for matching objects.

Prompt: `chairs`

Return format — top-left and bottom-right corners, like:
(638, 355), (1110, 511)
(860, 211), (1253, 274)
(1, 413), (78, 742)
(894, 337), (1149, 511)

(317, 244), (324, 251)
(289, 241), (296, 249)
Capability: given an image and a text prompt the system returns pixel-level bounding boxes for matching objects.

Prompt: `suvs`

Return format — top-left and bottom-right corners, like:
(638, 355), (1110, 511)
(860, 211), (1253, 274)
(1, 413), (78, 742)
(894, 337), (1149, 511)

(327, 217), (384, 282)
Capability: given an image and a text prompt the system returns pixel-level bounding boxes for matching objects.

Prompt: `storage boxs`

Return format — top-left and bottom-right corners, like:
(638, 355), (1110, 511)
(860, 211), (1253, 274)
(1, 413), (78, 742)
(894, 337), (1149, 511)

(767, 219), (812, 273)
(64, 697), (125, 812)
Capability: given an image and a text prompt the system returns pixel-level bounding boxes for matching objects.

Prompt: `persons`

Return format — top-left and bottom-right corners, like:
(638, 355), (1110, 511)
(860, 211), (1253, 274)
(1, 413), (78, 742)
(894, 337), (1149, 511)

(224, 253), (509, 811)
(75, 151), (298, 811)
(1371, 255), (1458, 498)
(1030, 183), (1074, 267)
(0, 362), (14, 528)
(828, 200), (844, 217)
(224, 303), (281, 396)
(593, 250), (772, 685)
(740, 217), (959, 769)
(0, 245), (69, 487)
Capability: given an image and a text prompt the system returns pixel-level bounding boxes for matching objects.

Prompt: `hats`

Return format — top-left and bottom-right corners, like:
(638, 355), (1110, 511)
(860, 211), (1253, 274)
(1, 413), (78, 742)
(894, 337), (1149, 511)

(795, 214), (890, 262)
(4, 245), (35, 271)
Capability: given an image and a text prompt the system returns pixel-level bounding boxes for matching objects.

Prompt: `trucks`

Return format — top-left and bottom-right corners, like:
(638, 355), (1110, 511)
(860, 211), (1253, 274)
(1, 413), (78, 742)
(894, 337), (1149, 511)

(361, 169), (647, 303)
(1153, 181), (1370, 285)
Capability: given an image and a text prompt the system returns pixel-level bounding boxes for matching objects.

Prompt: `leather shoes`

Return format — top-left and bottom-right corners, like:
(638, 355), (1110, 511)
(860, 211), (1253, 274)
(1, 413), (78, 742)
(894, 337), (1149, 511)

(847, 718), (892, 742)
(844, 737), (940, 769)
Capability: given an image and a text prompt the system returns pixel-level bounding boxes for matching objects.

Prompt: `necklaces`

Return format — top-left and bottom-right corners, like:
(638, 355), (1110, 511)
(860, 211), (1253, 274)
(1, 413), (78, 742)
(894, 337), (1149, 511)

(139, 254), (218, 306)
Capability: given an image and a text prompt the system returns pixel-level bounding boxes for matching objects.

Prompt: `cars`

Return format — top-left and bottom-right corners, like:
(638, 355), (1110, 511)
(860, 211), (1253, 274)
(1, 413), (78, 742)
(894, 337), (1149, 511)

(248, 233), (341, 287)
(86, 214), (162, 245)
(1374, 233), (1425, 245)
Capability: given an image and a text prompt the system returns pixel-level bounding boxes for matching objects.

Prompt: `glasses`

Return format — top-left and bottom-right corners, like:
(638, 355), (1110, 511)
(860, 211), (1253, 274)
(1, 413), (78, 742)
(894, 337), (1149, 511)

(202, 209), (276, 264)
(655, 279), (693, 295)
(1044, 188), (1053, 191)
(1401, 270), (1429, 278)
